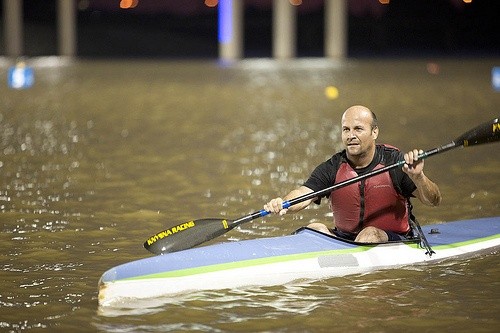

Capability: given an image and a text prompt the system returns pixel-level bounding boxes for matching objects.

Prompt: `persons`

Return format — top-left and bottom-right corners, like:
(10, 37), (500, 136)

(262, 105), (442, 243)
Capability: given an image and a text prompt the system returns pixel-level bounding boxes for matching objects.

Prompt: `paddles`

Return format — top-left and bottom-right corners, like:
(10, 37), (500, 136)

(143, 117), (500, 254)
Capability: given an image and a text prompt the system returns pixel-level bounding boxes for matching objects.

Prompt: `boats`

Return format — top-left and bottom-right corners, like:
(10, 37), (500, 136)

(97, 217), (500, 306)
